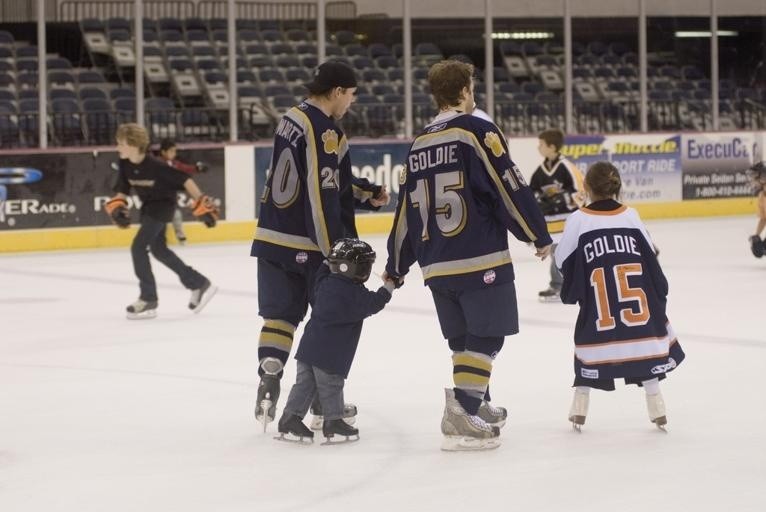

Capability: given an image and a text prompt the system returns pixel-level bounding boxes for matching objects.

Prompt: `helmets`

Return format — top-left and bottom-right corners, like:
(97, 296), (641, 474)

(327, 238), (377, 285)
(745, 161), (766, 190)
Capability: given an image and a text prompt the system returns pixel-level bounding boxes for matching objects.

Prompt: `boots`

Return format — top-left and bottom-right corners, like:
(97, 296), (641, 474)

(440, 388), (508, 439)
(567, 390), (590, 425)
(645, 392), (668, 425)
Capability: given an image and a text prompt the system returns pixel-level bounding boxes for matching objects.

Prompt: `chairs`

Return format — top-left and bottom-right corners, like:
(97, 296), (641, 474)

(0, 10), (227, 148)
(412, 30), (445, 133)
(236, 18), (405, 141)
(473, 38), (765, 136)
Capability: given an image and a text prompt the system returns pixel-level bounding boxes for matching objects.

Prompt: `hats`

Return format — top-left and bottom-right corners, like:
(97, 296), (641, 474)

(301, 61), (358, 94)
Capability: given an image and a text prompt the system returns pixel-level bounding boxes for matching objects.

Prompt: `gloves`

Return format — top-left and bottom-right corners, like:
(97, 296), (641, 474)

(749, 235), (764, 258)
(103, 198), (131, 229)
(189, 193), (219, 228)
(193, 160), (211, 173)
(542, 190), (571, 209)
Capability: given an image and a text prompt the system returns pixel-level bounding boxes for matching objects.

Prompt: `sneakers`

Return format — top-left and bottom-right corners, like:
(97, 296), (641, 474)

(176, 231), (186, 242)
(278, 413), (315, 438)
(126, 298), (158, 314)
(310, 402), (360, 438)
(188, 278), (211, 310)
(254, 373), (280, 423)
(538, 287), (560, 296)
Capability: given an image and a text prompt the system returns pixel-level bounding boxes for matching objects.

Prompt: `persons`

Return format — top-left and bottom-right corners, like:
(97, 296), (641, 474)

(276, 238), (404, 444)
(156, 140), (209, 243)
(378, 59), (550, 447)
(105, 124), (220, 320)
(745, 161), (766, 258)
(556, 163), (684, 436)
(529, 129), (587, 303)
(254, 56), (389, 433)
